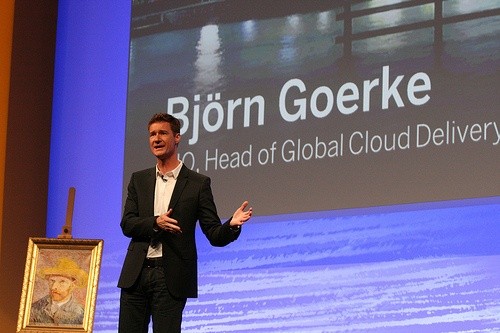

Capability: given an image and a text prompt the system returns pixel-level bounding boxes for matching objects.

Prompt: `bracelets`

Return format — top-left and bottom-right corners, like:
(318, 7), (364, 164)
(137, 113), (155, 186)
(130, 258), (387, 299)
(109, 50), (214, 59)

(233, 226), (240, 229)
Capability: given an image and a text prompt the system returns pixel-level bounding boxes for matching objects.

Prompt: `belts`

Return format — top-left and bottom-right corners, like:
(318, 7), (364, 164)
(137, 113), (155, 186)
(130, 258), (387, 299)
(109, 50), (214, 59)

(143, 258), (164, 267)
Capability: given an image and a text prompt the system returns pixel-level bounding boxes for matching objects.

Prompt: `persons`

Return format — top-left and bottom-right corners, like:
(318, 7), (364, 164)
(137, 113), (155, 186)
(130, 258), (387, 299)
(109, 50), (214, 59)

(117, 113), (252, 333)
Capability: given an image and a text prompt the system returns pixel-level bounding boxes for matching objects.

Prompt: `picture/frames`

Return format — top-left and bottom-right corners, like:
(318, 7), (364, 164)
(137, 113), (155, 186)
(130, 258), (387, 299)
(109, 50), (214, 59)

(16, 237), (104, 333)
